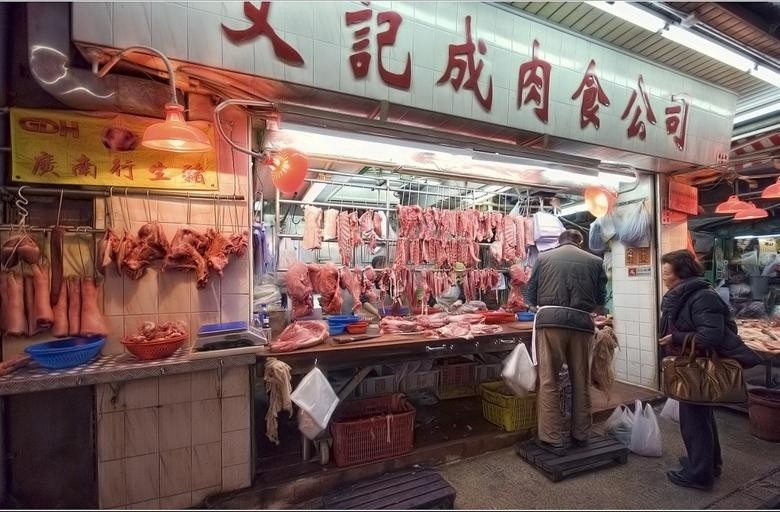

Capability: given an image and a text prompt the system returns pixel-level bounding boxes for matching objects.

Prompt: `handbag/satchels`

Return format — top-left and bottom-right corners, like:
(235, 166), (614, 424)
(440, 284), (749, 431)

(661, 333), (750, 407)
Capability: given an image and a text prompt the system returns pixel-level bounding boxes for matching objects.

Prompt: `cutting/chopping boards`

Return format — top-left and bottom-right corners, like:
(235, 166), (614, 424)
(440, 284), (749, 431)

(328, 334), (410, 346)
(508, 323), (533, 330)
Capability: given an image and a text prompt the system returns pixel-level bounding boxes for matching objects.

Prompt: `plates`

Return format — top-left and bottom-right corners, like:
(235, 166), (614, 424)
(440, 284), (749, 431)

(326, 316), (360, 322)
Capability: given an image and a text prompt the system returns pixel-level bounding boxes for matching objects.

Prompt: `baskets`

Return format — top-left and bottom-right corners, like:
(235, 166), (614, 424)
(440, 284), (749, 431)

(25, 337), (102, 368)
(120, 333), (186, 359)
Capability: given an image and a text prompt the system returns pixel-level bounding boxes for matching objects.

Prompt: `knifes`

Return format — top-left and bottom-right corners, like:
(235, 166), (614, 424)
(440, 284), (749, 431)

(339, 334), (380, 344)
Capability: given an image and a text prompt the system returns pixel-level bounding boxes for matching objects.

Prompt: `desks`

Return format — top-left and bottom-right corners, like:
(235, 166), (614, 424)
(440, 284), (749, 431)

(254, 310), (612, 462)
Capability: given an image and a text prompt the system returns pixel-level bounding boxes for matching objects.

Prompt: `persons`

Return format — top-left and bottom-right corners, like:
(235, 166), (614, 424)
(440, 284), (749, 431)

(662, 249), (765, 493)
(523, 228), (608, 457)
(352, 256), (396, 324)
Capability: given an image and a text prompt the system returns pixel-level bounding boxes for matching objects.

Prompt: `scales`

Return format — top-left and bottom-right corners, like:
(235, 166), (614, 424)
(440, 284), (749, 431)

(193, 320), (267, 349)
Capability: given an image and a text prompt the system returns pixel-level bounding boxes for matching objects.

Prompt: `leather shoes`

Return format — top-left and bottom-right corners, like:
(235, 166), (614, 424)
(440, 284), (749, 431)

(678, 455), (722, 478)
(667, 469), (716, 491)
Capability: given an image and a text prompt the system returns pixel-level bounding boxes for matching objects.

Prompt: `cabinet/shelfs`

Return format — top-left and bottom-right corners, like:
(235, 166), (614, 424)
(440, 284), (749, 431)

(275, 170), (557, 316)
(0, 182), (252, 397)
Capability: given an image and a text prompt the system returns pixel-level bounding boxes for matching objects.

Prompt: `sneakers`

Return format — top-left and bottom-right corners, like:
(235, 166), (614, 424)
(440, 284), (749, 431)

(533, 439), (567, 456)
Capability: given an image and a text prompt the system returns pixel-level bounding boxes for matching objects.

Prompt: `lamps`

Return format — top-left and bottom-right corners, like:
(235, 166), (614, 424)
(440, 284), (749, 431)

(91, 45), (212, 156)
(734, 185), (769, 221)
(584, 166), (641, 218)
(715, 181), (752, 214)
(762, 175), (780, 199)
(213, 98), (311, 198)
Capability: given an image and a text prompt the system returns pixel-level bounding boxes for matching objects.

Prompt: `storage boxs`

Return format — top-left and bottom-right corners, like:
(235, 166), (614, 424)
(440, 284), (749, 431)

(483, 380), (536, 431)
(331, 396), (416, 465)
